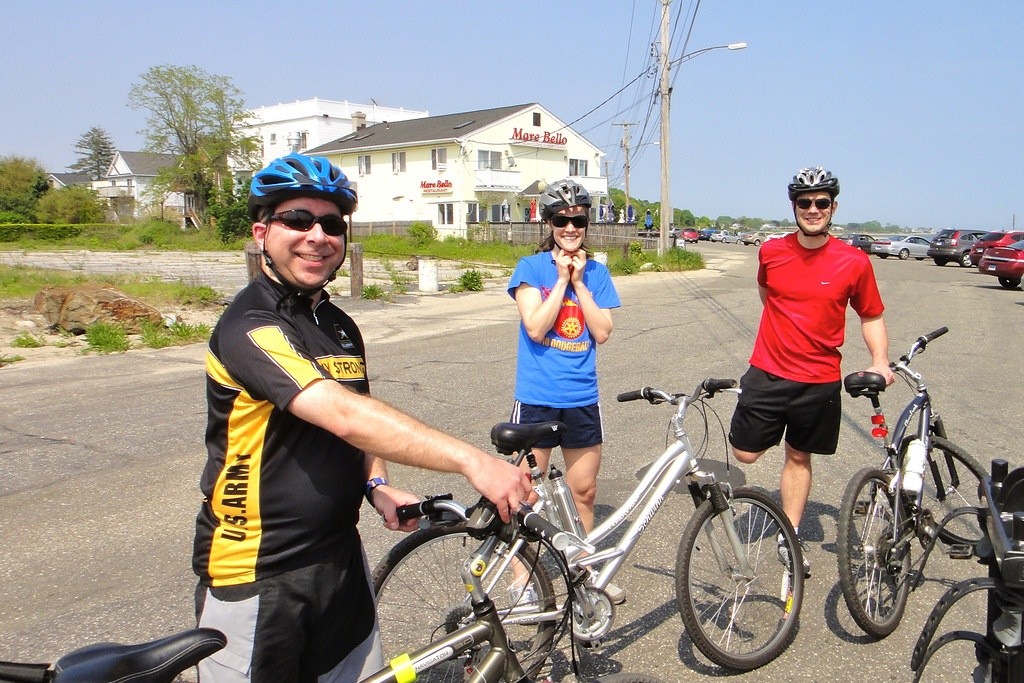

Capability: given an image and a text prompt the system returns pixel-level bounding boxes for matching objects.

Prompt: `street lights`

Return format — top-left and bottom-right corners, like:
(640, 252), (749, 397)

(624, 141), (661, 223)
(655, 41), (747, 260)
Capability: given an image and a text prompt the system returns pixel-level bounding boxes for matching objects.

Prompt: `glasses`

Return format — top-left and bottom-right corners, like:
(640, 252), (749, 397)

(795, 199), (833, 209)
(550, 215), (588, 228)
(264, 209), (348, 236)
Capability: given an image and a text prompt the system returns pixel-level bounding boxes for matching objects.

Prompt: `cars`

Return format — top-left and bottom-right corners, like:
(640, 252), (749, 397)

(764, 232), (794, 243)
(837, 233), (876, 254)
(927, 229), (991, 268)
(978, 239), (1024, 291)
(669, 226), (742, 245)
(968, 229), (1024, 271)
(871, 234), (933, 261)
(739, 231), (774, 247)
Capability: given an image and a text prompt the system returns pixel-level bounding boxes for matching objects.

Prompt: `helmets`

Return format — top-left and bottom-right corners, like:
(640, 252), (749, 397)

(247, 152), (357, 222)
(538, 179), (592, 221)
(788, 166), (840, 201)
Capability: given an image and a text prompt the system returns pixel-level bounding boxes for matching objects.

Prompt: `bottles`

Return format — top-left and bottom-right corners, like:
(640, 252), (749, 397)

(902, 436), (926, 496)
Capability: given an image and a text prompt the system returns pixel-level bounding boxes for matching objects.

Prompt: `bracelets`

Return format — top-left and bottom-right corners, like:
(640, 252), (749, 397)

(364, 477), (388, 508)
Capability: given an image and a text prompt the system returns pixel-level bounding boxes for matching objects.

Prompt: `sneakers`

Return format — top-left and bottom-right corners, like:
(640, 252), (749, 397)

(775, 529), (811, 575)
(588, 571), (626, 604)
(511, 583), (541, 625)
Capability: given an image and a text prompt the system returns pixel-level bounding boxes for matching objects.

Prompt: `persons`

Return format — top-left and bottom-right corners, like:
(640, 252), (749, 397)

(192, 152), (533, 683)
(508, 180), (626, 611)
(729, 168), (897, 574)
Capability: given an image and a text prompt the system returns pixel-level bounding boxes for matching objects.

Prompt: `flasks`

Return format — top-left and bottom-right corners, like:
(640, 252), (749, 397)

(548, 464), (587, 541)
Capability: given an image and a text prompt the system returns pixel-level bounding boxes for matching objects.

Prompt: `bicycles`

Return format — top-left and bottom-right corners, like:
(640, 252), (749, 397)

(369, 377), (806, 683)
(836, 326), (995, 637)
(0, 493), (666, 683)
